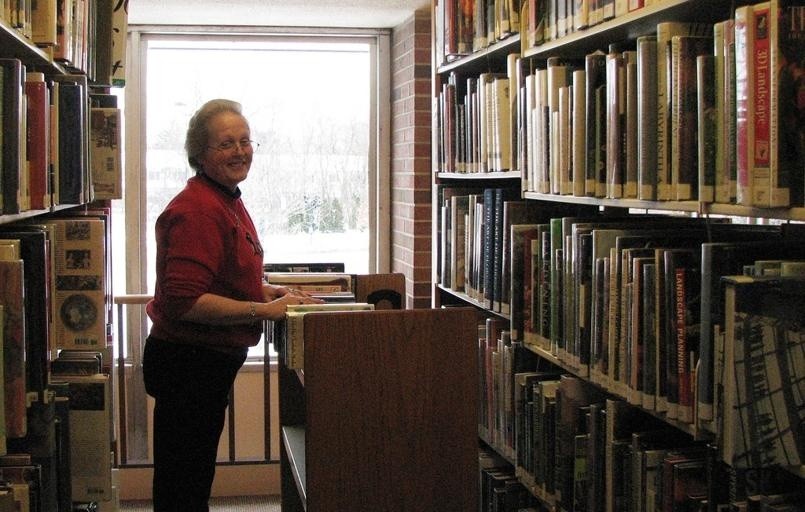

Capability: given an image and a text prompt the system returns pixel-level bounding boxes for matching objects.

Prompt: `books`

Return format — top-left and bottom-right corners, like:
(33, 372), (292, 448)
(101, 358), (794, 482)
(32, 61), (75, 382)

(429, 1), (804, 511)
(1, 2), (137, 512)
(263, 270), (376, 371)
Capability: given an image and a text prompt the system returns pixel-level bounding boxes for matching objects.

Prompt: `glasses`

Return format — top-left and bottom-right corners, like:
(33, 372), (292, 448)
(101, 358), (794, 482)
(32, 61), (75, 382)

(207, 140), (260, 156)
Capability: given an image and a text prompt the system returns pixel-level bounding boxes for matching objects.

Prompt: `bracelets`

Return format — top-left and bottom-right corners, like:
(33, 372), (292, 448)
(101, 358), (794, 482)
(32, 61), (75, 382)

(248, 300), (256, 323)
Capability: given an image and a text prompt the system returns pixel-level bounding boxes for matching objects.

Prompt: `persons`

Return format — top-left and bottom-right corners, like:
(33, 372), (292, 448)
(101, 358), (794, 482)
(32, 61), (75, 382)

(142, 99), (324, 511)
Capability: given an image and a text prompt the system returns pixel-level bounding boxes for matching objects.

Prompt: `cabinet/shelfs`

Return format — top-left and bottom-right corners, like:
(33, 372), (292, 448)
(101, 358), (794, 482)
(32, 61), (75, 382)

(0, 0), (111, 512)
(279, 271), (481, 512)
(432, 0), (804, 512)
